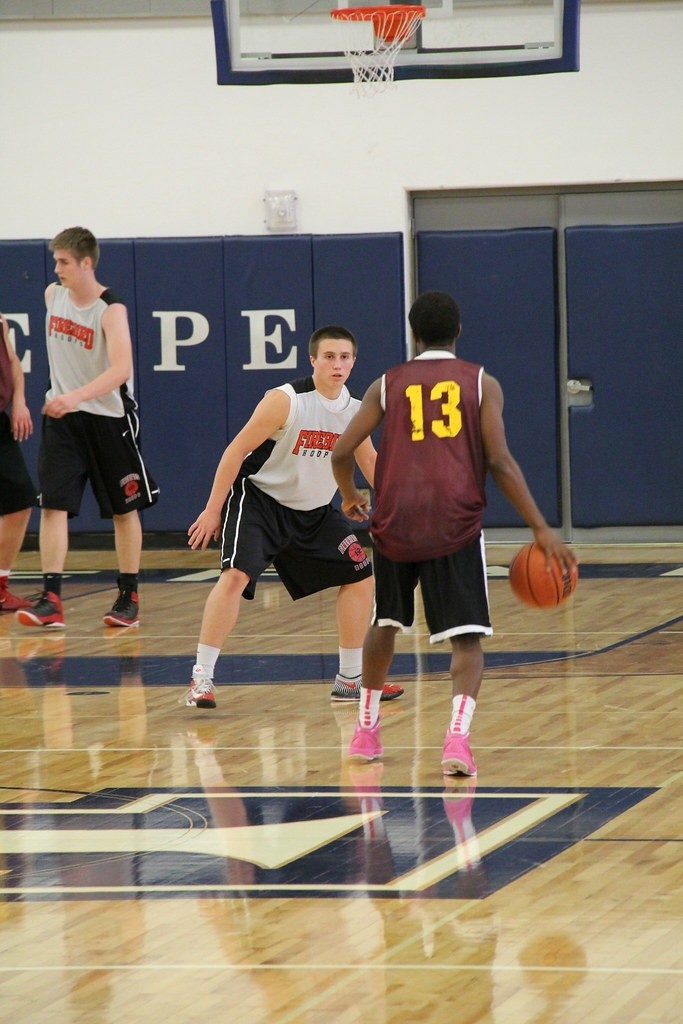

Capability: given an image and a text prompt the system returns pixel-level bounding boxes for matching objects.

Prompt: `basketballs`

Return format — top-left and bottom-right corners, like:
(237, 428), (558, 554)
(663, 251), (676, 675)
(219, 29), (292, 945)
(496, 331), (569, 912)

(507, 541), (581, 609)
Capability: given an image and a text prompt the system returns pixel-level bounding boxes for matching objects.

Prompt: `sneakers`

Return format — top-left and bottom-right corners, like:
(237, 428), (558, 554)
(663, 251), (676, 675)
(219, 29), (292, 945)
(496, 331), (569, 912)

(443, 775), (477, 824)
(442, 724), (477, 776)
(349, 763), (384, 807)
(185, 672), (216, 708)
(103, 585), (140, 627)
(331, 673), (404, 701)
(0, 576), (32, 614)
(348, 714), (382, 761)
(17, 631), (65, 672)
(16, 580), (65, 627)
(331, 702), (405, 727)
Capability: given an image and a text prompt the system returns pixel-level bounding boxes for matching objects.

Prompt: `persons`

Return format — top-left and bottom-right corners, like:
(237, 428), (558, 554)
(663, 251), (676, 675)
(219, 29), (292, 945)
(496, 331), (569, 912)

(184, 325), (405, 708)
(332, 290), (575, 774)
(0, 313), (37, 608)
(185, 716), (354, 1024)
(15, 227), (158, 626)
(15, 627), (147, 1012)
(353, 774), (566, 1024)
